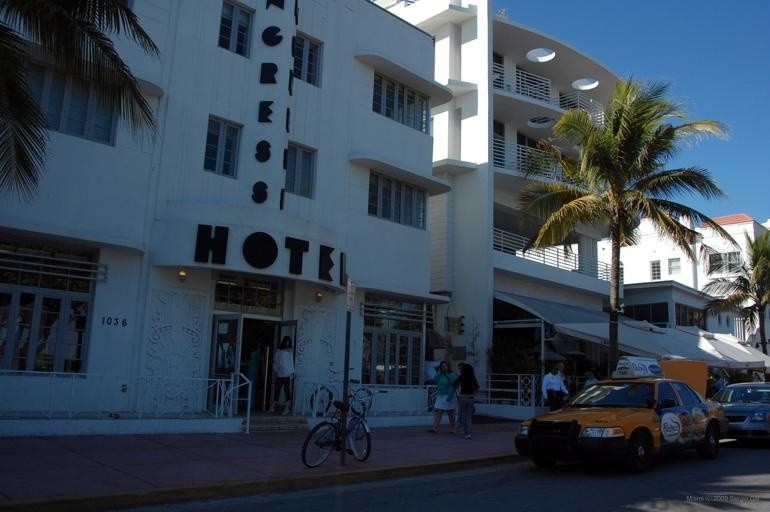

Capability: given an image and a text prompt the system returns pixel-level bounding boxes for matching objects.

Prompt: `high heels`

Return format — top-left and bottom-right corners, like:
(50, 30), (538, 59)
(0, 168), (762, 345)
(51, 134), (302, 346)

(265, 409), (275, 416)
(280, 409), (290, 417)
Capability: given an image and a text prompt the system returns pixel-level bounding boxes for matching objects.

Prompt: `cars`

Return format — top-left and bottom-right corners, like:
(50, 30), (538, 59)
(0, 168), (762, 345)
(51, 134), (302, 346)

(710, 382), (770, 443)
(514, 356), (730, 474)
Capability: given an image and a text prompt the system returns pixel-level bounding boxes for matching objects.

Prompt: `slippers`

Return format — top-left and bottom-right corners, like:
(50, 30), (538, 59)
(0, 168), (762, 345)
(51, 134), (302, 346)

(427, 429), (438, 433)
(448, 429), (457, 434)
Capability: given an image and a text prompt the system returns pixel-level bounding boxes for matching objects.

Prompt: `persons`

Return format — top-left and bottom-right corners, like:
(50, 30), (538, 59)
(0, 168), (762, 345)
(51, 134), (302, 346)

(713, 369), (729, 391)
(541, 362), (570, 411)
(452, 364), (481, 439)
(457, 362), (465, 373)
(267, 336), (294, 417)
(583, 371), (599, 388)
(429, 361), (459, 435)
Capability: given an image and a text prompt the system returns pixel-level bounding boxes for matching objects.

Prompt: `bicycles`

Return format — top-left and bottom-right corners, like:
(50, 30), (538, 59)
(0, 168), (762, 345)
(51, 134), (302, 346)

(310, 360), (373, 416)
(301, 390), (388, 468)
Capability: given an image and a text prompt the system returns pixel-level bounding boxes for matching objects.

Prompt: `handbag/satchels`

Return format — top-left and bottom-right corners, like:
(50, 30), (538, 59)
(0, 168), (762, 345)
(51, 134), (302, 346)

(547, 389), (566, 405)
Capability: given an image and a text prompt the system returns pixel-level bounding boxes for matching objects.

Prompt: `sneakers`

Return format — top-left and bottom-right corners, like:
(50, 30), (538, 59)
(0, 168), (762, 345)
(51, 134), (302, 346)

(464, 434), (471, 439)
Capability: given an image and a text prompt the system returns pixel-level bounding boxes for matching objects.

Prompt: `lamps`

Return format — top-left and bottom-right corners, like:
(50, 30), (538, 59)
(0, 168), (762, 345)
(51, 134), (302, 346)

(315, 291), (323, 303)
(178, 268), (188, 282)
